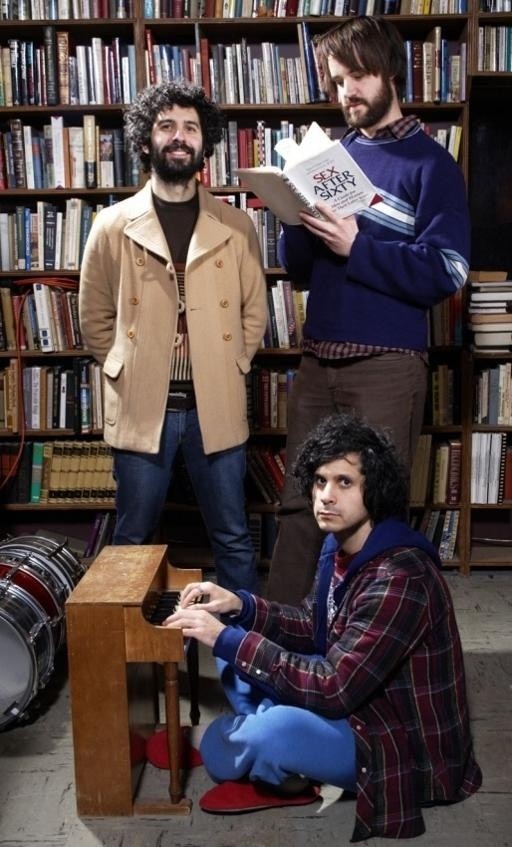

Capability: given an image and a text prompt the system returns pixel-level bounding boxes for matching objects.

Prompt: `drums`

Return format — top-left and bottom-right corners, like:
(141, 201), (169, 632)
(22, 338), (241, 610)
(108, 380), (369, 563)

(0, 535), (85, 726)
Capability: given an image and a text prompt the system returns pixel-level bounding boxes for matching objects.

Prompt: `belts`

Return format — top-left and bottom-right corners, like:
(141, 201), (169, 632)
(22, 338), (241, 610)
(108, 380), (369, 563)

(167, 395), (197, 410)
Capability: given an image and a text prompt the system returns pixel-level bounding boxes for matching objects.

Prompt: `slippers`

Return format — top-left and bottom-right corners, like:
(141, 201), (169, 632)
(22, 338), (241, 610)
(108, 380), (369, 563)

(199, 779), (321, 815)
(144, 726), (204, 769)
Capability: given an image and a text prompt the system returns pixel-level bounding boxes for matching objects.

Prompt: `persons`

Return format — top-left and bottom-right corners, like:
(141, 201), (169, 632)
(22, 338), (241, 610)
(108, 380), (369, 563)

(79, 79), (269, 591)
(144, 412), (483, 840)
(266, 13), (471, 601)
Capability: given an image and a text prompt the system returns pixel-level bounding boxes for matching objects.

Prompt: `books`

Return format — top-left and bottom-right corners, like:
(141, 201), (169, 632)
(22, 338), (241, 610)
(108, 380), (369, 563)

(0, 284), (116, 503)
(244, 366), (462, 561)
(1, 0), (138, 272)
(477, 1), (512, 71)
(144, 0), (468, 351)
(471, 272), (511, 353)
(472, 363), (511, 505)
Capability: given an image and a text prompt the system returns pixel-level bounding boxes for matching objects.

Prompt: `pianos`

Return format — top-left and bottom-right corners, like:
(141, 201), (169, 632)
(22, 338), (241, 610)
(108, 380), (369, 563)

(65, 545), (204, 817)
